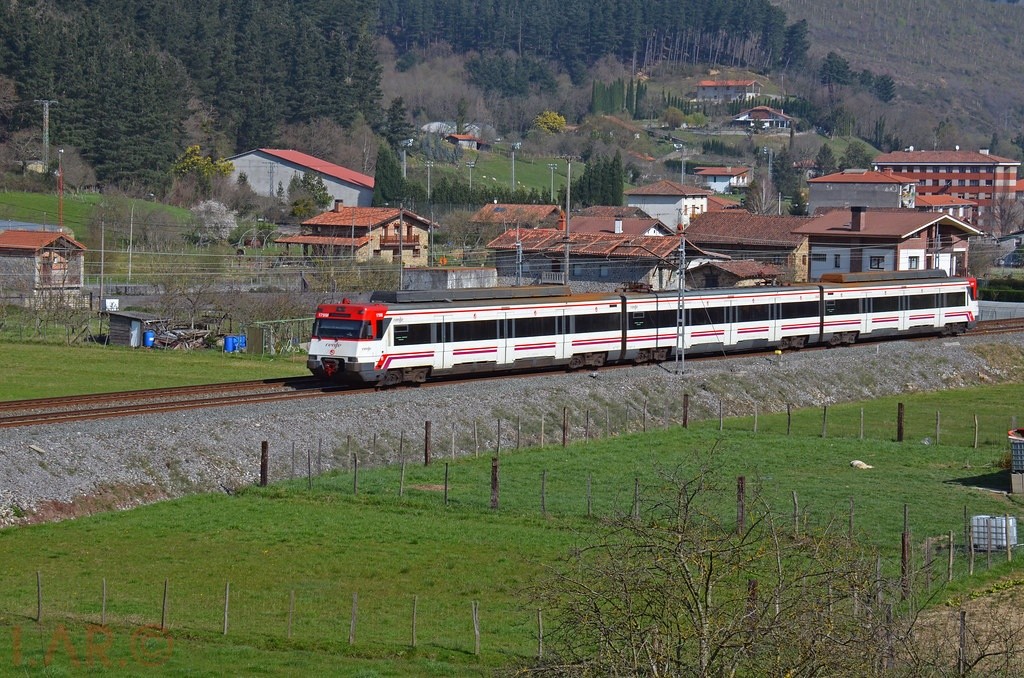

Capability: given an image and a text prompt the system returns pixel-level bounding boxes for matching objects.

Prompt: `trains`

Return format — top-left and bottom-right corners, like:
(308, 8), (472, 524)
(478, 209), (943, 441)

(305, 269), (981, 389)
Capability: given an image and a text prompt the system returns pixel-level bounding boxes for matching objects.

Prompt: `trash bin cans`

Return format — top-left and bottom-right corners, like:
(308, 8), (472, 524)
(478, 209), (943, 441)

(239, 336), (246, 348)
(225, 336), (234, 351)
(233, 336), (241, 350)
(143, 331), (153, 347)
